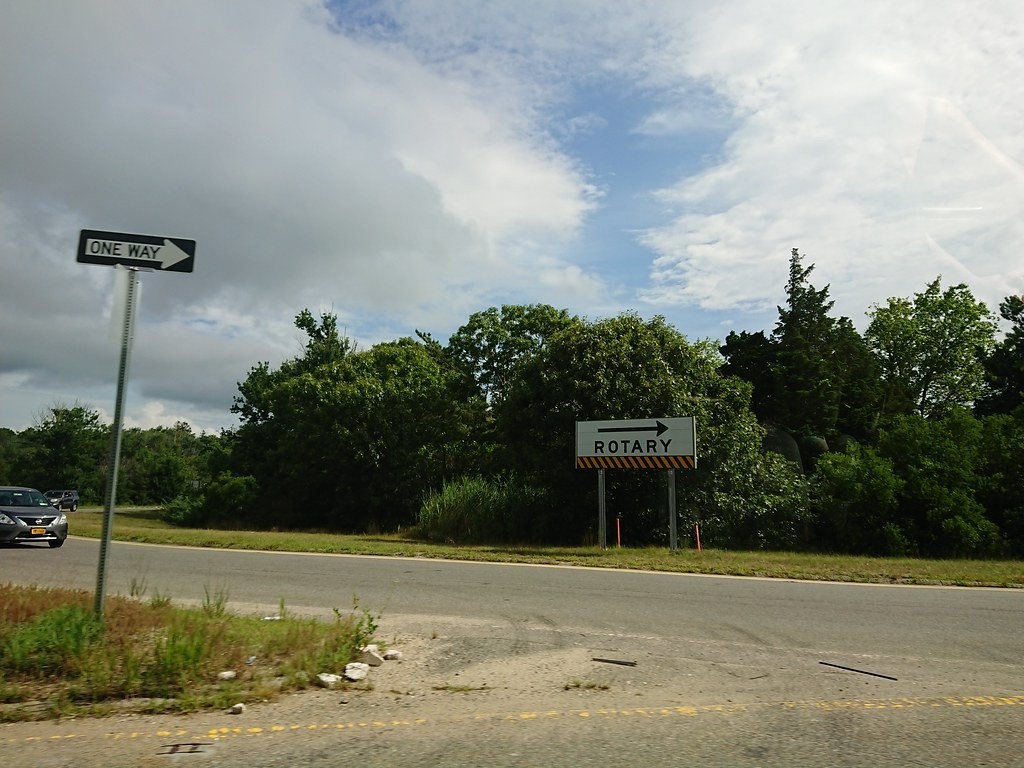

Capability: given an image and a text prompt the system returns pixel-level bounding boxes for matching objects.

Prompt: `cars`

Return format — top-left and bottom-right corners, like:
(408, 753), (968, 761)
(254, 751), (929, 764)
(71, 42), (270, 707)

(0, 485), (69, 548)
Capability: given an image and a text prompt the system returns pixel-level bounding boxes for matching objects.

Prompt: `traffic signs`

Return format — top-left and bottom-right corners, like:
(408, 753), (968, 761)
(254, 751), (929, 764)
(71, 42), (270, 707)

(74, 227), (197, 276)
(572, 415), (700, 471)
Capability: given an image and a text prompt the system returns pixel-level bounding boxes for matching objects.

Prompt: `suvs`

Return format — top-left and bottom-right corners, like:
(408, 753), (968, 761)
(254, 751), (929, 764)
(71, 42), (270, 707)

(43, 489), (80, 512)
